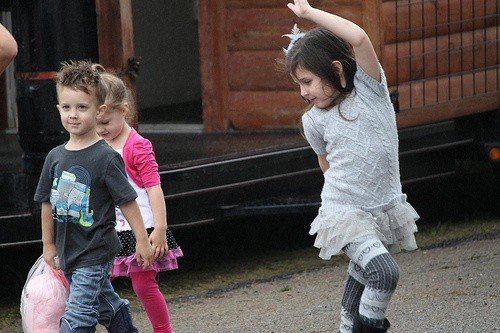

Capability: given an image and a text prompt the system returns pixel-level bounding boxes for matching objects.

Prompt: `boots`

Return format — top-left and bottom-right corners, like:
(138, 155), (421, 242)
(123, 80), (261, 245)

(59, 317), (98, 333)
(102, 302), (138, 333)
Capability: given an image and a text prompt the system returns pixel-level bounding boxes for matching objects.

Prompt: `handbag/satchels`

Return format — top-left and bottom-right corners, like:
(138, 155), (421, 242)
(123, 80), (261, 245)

(18, 254), (71, 333)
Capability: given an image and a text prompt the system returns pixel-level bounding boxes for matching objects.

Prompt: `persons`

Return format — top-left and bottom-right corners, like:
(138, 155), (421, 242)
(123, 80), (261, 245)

(89, 64), (183, 333)
(33, 60), (154, 333)
(0, 23), (18, 75)
(282, 0), (420, 333)
